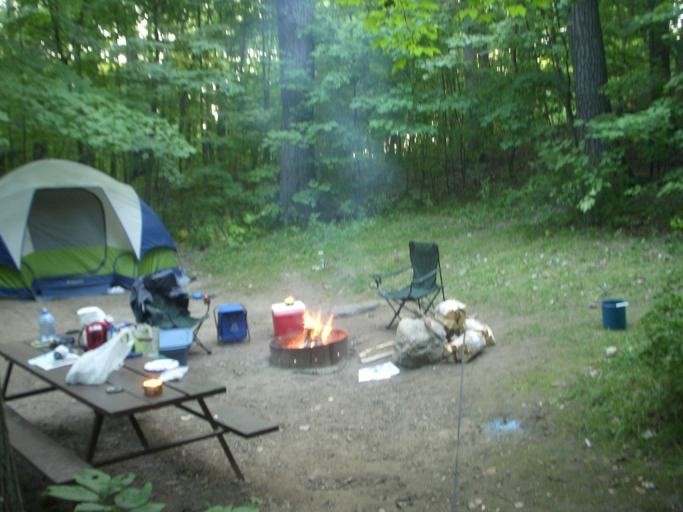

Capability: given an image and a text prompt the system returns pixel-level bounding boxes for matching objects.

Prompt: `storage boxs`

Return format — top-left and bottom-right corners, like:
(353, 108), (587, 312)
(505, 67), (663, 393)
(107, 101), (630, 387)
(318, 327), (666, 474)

(271, 300), (306, 335)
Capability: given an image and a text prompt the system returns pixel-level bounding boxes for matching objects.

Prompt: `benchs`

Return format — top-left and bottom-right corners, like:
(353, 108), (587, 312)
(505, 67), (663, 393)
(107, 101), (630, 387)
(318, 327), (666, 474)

(178, 398), (279, 440)
(0, 405), (95, 486)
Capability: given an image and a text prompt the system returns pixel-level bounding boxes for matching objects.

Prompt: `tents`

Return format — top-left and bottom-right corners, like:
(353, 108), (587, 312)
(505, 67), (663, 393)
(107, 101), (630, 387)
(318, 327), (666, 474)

(0, 158), (183, 302)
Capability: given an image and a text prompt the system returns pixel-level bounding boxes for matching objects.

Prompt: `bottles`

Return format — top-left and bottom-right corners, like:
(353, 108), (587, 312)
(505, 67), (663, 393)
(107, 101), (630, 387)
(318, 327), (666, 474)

(191, 276), (202, 300)
(54, 336), (75, 360)
(39, 309), (57, 344)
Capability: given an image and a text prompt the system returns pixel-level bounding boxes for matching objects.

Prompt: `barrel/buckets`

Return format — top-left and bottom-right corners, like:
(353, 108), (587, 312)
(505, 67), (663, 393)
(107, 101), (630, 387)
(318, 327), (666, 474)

(37, 307), (58, 343)
(600, 299), (631, 331)
(85, 318), (111, 350)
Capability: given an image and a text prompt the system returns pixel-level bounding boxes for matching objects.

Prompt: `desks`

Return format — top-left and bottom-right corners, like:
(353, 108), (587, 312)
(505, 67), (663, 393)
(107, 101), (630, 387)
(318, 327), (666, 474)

(0, 333), (246, 485)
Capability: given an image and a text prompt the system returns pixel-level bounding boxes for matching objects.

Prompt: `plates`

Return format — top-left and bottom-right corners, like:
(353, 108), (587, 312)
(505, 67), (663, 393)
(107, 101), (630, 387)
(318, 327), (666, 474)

(143, 358), (179, 371)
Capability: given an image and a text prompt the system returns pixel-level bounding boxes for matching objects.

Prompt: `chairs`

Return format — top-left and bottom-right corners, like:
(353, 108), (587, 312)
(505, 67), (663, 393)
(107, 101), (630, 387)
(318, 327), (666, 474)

(376, 240), (444, 331)
(129, 269), (212, 355)
